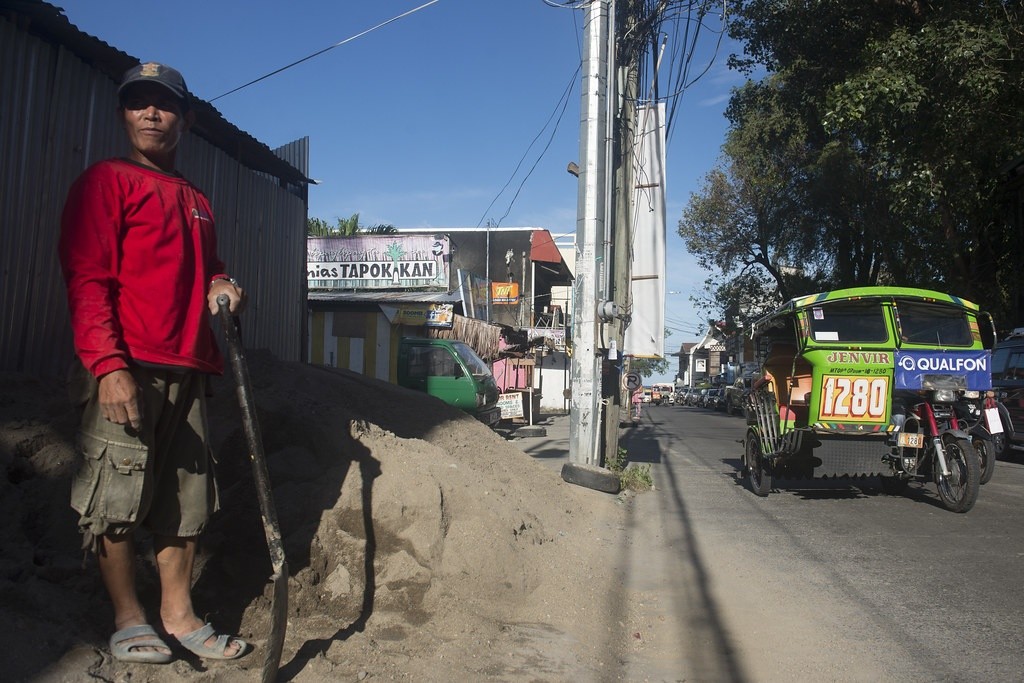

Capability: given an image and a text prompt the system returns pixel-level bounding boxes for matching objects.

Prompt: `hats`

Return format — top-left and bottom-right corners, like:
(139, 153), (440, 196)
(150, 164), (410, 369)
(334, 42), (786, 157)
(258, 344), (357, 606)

(118, 62), (190, 104)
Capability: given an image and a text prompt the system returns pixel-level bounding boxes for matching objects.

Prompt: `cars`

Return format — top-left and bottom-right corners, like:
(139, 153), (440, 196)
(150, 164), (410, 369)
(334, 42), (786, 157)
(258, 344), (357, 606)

(675, 377), (752, 417)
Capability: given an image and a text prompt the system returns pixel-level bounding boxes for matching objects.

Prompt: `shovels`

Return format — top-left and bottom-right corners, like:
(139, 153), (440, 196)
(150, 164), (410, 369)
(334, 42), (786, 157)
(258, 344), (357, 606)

(216, 293), (290, 683)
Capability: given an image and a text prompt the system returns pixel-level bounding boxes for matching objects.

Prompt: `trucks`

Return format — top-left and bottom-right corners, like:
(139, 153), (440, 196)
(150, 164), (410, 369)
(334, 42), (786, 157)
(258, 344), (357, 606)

(398, 338), (502, 425)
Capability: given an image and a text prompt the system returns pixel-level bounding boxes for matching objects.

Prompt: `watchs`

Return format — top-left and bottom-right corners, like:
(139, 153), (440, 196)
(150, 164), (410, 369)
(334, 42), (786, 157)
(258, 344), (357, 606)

(209, 276), (240, 288)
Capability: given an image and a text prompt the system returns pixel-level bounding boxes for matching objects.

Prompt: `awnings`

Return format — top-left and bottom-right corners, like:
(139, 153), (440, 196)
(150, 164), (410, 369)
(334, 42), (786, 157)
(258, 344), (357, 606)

(530, 229), (574, 286)
(307, 289), (462, 301)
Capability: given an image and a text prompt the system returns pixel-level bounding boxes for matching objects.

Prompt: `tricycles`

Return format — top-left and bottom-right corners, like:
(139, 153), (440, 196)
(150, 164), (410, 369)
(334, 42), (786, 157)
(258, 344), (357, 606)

(745, 286), (1024, 513)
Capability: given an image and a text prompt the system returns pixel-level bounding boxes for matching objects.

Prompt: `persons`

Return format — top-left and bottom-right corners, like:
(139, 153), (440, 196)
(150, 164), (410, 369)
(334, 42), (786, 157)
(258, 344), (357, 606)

(632, 384), (643, 419)
(71, 62), (248, 660)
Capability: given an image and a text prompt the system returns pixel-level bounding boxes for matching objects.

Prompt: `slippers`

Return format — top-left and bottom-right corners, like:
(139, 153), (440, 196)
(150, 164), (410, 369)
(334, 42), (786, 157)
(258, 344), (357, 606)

(108, 624), (175, 664)
(169, 622), (246, 660)
